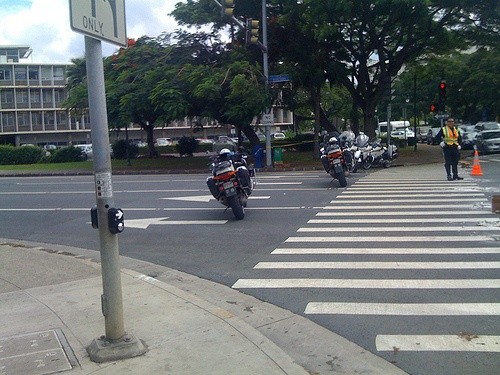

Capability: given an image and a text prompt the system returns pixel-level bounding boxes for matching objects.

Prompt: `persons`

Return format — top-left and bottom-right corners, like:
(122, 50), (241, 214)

(435, 117), (464, 181)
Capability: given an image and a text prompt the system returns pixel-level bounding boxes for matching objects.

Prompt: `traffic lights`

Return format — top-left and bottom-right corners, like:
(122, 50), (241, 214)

(430, 103), (436, 112)
(438, 81), (446, 104)
(220, 0), (234, 19)
(246, 17), (260, 44)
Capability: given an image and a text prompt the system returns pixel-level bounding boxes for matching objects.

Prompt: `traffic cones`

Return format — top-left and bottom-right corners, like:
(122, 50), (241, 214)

(469, 150), (484, 176)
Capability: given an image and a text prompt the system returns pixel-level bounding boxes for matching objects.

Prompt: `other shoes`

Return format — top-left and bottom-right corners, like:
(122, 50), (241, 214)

(447, 175), (453, 181)
(453, 174), (464, 180)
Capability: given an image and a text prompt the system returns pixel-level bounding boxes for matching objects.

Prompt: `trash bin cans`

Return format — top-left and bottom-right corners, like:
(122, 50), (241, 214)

(252, 144), (264, 168)
(272, 146), (282, 162)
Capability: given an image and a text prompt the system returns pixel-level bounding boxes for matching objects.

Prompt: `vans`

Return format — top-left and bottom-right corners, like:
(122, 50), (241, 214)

(377, 119), (416, 147)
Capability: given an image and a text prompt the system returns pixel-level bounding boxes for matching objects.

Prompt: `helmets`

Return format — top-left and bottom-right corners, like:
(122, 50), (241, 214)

(329, 136), (338, 143)
(219, 148), (231, 156)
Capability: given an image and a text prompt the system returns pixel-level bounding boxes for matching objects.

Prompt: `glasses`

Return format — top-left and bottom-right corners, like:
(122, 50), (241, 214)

(448, 121), (454, 122)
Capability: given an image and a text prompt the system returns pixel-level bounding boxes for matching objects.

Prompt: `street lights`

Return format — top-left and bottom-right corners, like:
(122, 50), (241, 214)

(412, 57), (430, 152)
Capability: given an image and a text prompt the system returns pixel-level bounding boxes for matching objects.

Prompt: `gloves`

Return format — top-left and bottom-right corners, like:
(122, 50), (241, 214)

(440, 141), (446, 148)
(457, 145), (461, 151)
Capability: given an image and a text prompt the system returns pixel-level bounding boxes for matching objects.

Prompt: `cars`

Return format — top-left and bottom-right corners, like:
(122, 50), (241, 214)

(19, 143), (93, 162)
(410, 120), (500, 156)
(382, 131), (408, 148)
(269, 132), (286, 139)
(129, 133), (239, 150)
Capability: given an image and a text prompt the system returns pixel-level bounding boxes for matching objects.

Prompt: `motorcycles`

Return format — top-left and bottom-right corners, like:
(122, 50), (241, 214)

(205, 148), (255, 221)
(318, 130), (399, 188)
